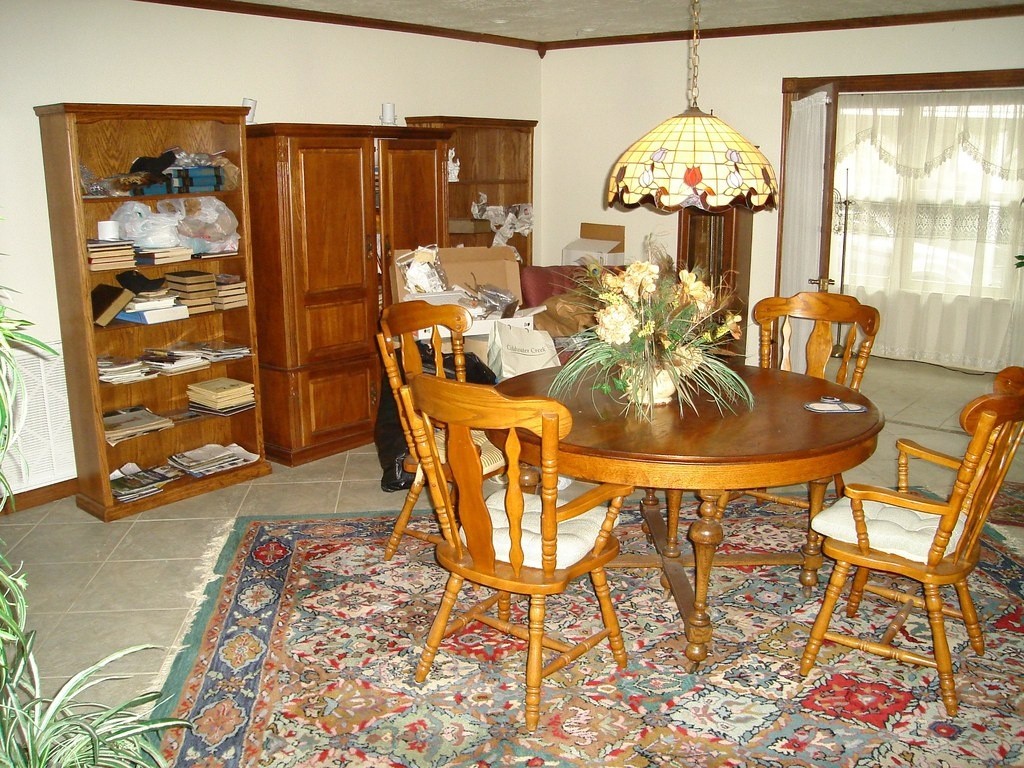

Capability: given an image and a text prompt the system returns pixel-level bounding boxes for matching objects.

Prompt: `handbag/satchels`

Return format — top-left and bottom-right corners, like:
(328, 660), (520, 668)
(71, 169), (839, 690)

(485, 318), (560, 384)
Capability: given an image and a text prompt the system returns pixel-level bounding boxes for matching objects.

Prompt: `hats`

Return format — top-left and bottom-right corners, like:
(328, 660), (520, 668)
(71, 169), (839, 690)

(129, 151), (176, 174)
(116, 270), (166, 294)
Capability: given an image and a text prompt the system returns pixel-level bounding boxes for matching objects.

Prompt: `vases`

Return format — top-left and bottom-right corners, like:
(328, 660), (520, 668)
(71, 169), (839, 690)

(618, 361), (686, 405)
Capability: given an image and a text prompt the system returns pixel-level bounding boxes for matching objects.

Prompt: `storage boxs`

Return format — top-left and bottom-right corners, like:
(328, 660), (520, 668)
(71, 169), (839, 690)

(388, 247), (548, 340)
(562, 223), (626, 266)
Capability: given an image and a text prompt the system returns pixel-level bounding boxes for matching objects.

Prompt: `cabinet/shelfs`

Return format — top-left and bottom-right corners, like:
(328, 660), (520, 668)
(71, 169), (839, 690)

(405, 116), (539, 266)
(245, 124), (456, 469)
(36, 102), (272, 524)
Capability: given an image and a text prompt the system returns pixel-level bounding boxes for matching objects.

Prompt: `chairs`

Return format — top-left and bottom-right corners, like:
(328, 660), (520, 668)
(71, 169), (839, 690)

(400, 374), (636, 733)
(374, 300), (535, 592)
(715, 292), (880, 547)
(799, 363), (1024, 717)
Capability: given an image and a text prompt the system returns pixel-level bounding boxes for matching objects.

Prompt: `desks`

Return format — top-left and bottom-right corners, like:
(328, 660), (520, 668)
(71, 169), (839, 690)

(485, 362), (887, 664)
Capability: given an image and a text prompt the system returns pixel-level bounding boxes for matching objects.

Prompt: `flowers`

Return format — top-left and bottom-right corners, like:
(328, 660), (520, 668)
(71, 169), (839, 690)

(545, 234), (760, 423)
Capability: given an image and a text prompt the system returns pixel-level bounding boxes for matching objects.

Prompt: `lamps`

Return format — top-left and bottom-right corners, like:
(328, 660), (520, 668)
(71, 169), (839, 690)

(608, 0), (781, 213)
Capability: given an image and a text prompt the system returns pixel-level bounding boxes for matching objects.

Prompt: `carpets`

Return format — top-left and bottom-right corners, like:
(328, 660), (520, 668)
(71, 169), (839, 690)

(120, 475), (1024, 768)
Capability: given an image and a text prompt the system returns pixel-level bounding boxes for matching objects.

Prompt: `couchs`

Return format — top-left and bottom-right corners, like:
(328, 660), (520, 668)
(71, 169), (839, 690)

(518, 266), (630, 366)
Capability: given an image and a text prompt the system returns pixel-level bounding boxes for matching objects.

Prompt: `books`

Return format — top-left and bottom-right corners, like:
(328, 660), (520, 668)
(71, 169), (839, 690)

(97, 334), (255, 384)
(102, 376), (260, 505)
(85, 239), (250, 326)
